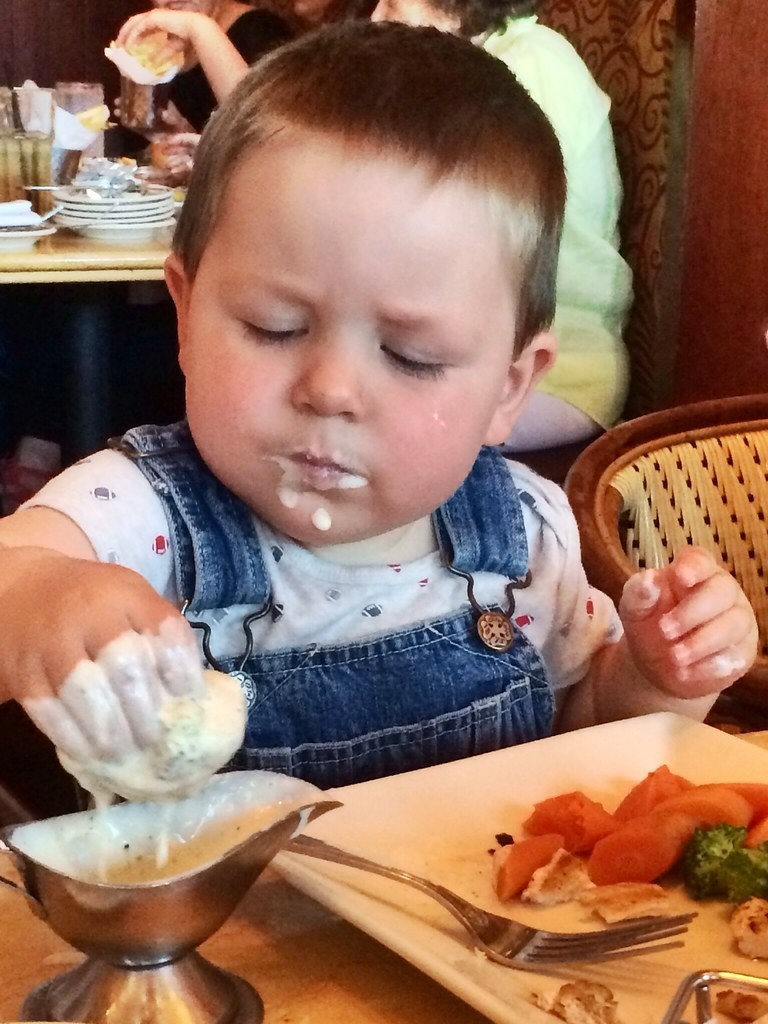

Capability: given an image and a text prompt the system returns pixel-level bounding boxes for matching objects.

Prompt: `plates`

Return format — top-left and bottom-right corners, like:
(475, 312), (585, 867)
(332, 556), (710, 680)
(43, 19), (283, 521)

(50, 183), (185, 239)
(267, 711), (768, 1024)
(0, 222), (56, 252)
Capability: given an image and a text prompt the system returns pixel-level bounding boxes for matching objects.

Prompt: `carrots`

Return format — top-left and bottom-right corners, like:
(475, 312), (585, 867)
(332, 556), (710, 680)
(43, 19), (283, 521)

(498, 768), (768, 904)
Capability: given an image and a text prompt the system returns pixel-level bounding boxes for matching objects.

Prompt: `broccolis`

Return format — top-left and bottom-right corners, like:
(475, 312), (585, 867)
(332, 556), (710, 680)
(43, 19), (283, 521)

(682, 824), (768, 902)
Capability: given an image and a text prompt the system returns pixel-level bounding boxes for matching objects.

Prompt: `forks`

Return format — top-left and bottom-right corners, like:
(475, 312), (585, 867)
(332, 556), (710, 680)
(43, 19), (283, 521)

(281, 833), (698, 970)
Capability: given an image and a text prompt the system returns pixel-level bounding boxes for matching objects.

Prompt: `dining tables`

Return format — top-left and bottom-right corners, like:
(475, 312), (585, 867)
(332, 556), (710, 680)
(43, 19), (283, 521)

(3, 726), (768, 1023)
(0, 184), (187, 467)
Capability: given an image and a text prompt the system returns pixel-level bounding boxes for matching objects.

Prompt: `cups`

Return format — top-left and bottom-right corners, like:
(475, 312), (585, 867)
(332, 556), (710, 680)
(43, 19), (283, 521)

(0, 82), (106, 220)
(120, 75), (170, 131)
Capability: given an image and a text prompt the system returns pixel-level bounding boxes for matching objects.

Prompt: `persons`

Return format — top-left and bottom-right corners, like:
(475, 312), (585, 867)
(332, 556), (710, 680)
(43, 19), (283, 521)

(51, 0), (635, 458)
(0, 20), (757, 800)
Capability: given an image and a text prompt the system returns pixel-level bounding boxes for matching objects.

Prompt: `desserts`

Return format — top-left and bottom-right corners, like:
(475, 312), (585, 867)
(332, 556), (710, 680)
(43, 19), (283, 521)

(110, 37), (179, 75)
(54, 664), (247, 874)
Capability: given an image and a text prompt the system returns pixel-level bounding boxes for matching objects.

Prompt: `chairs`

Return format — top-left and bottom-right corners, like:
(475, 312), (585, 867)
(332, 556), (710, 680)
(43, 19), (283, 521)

(563, 394), (768, 735)
